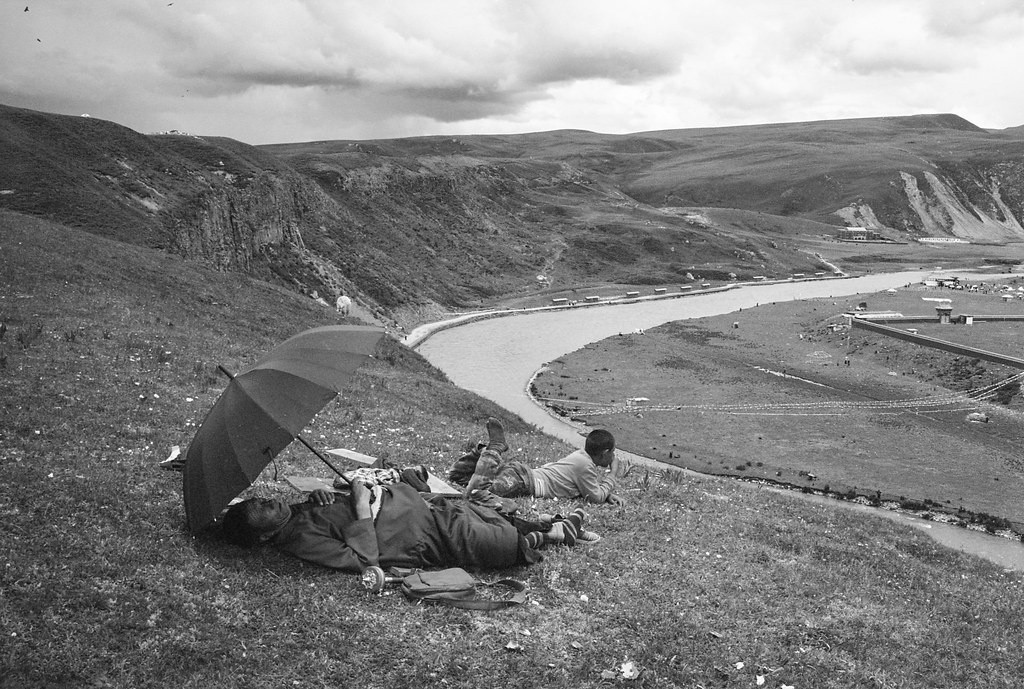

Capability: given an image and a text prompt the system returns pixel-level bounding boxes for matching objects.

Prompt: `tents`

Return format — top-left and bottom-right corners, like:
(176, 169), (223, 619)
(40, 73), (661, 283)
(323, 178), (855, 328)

(887, 288), (897, 296)
(1002, 286), (1024, 302)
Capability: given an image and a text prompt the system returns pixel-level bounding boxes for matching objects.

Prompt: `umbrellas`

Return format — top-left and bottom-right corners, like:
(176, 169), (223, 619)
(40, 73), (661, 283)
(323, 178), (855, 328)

(183, 327), (388, 531)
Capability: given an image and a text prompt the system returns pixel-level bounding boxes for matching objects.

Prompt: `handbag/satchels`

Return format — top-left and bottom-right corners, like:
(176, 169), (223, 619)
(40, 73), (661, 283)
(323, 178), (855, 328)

(401, 567), (478, 604)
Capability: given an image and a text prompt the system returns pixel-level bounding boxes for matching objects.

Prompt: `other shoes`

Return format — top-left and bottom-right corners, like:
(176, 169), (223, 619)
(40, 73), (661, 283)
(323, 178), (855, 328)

(539, 512), (568, 524)
(576, 525), (601, 545)
(469, 488), (519, 516)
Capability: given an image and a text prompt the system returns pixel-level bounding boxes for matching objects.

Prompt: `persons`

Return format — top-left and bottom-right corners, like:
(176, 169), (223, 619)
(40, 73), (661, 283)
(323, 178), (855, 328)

(450, 417), (627, 506)
(223, 483), (601, 574)
